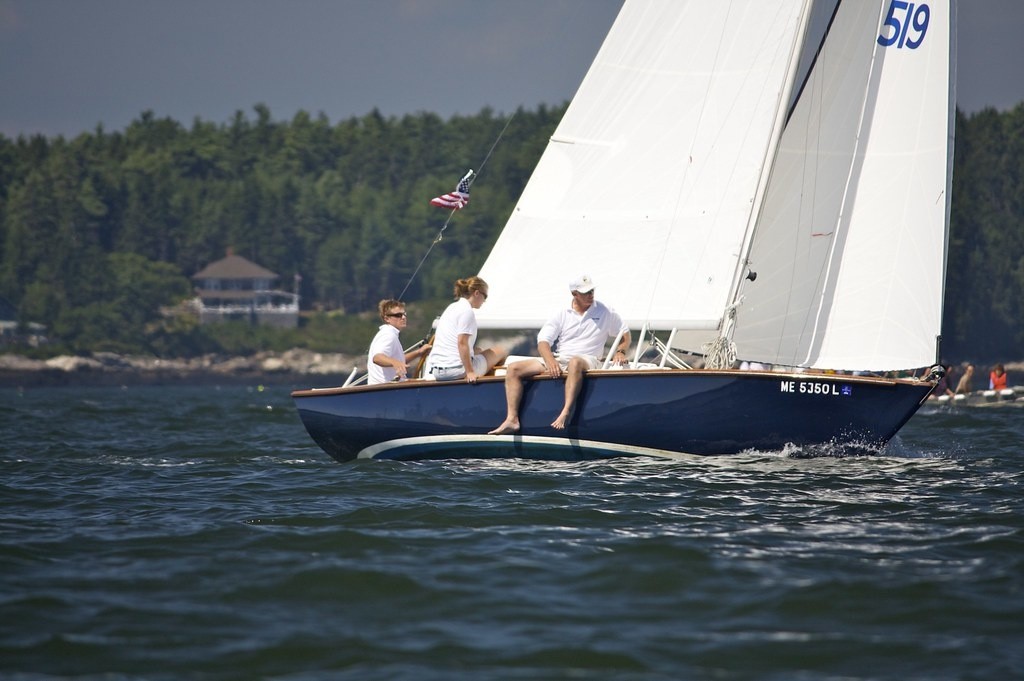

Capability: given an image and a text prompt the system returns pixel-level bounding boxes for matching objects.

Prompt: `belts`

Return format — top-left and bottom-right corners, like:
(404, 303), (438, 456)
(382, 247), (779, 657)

(435, 367), (445, 369)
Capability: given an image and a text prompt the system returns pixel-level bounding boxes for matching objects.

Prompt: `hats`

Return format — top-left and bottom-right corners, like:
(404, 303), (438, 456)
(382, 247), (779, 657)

(570, 275), (594, 294)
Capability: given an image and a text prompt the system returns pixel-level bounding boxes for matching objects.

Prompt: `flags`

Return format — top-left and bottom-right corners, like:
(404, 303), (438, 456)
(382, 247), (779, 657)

(427, 169), (474, 210)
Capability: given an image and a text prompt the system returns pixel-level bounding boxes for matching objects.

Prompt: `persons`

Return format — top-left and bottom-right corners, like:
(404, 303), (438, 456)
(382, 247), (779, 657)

(367, 299), (432, 386)
(488, 275), (631, 436)
(933, 361), (970, 397)
(428, 276), (509, 382)
(989, 363), (1008, 390)
(955, 363), (976, 394)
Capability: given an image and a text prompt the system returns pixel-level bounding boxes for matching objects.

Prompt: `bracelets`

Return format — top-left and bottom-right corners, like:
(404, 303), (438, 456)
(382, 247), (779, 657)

(616, 349), (626, 355)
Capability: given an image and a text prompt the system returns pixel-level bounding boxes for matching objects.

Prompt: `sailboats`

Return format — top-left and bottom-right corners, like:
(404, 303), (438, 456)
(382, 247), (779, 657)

(290, 0), (961, 462)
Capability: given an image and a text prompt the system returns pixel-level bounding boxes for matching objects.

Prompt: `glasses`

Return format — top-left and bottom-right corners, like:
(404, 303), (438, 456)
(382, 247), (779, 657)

(478, 291), (487, 299)
(586, 289), (593, 294)
(386, 312), (407, 318)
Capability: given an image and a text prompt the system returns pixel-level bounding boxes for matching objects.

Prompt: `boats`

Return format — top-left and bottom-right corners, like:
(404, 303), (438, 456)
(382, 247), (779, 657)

(925, 394), (967, 414)
(983, 387), (1024, 403)
